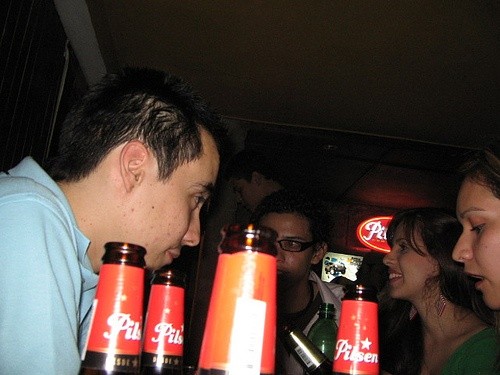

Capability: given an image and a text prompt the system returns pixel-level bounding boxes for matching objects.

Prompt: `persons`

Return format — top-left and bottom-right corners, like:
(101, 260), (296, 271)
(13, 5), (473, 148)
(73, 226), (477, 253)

(0, 63), (220, 375)
(452, 149), (500, 309)
(383, 207), (500, 375)
(329, 250), (389, 300)
(239, 185), (345, 375)
(226, 148), (288, 211)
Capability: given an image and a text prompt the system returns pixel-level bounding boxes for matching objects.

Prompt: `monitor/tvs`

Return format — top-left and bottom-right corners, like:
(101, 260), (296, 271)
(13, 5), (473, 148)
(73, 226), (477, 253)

(316, 245), (370, 283)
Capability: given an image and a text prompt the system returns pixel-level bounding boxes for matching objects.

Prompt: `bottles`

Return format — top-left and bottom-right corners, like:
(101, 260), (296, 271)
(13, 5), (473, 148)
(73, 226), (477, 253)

(75, 241), (148, 375)
(140, 265), (189, 375)
(278, 317), (331, 375)
(303, 303), (339, 374)
(331, 283), (381, 375)
(194, 223), (279, 375)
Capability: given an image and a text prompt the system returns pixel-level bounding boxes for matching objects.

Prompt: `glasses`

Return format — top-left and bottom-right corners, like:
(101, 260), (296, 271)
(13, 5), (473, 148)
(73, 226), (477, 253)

(263, 238), (313, 252)
